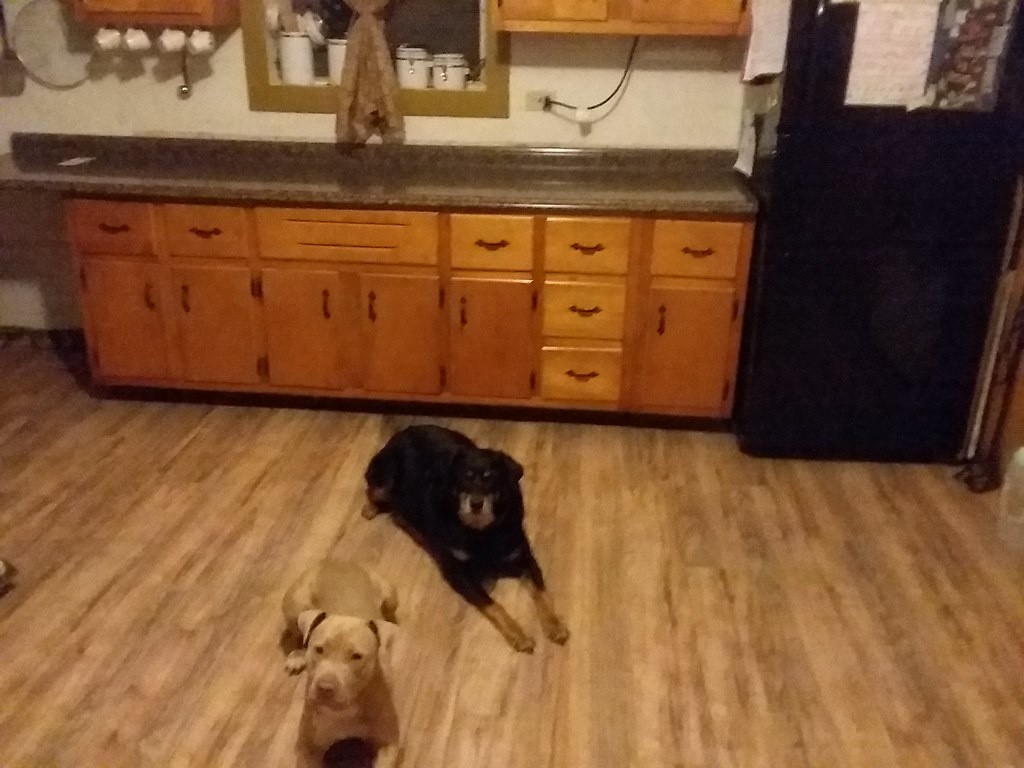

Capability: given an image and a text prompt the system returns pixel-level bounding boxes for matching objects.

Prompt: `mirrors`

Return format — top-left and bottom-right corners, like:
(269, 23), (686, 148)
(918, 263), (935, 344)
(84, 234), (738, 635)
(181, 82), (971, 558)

(240, 0), (510, 120)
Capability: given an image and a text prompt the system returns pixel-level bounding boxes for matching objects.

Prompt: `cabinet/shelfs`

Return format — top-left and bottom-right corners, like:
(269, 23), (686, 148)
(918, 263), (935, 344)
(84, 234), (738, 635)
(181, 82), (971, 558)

(445, 209), (537, 405)
(72, 0), (238, 30)
(255, 199), (444, 412)
(726, 0), (1024, 464)
(538, 213), (633, 408)
(635, 214), (753, 420)
(489, 1), (754, 38)
(69, 195), (254, 400)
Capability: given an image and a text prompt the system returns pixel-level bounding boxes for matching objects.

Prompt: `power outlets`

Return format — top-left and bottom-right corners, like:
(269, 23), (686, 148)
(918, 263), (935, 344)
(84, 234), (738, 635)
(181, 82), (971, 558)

(525, 89), (557, 111)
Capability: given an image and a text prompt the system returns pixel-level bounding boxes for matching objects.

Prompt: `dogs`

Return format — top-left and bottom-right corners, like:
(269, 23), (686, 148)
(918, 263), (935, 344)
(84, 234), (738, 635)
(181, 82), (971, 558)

(360, 425), (570, 655)
(282, 554), (401, 768)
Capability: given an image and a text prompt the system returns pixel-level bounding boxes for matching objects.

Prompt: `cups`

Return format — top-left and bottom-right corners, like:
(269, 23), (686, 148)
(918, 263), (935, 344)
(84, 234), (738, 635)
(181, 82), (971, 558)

(157, 28), (186, 52)
(278, 33), (315, 86)
(397, 46), (433, 90)
(327, 39), (350, 86)
(431, 55), (470, 93)
(186, 29), (217, 56)
(122, 28), (150, 52)
(95, 27), (121, 52)
